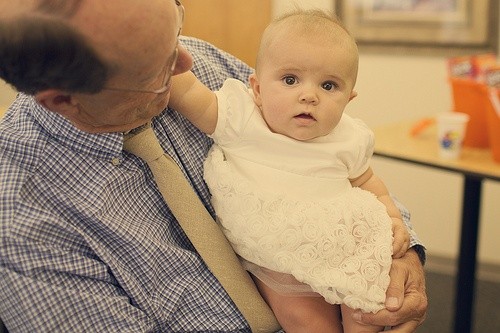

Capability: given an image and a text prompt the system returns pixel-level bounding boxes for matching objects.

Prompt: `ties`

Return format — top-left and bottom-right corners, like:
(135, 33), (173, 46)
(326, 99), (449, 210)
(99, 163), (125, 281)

(123, 120), (283, 333)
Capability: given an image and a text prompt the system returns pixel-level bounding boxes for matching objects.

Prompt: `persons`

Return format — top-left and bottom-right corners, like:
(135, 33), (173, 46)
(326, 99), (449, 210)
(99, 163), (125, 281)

(163, 9), (411, 333)
(0, 0), (429, 333)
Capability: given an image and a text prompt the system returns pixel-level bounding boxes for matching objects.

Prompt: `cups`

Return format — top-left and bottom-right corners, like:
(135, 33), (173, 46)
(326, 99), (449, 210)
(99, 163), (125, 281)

(436, 111), (469, 159)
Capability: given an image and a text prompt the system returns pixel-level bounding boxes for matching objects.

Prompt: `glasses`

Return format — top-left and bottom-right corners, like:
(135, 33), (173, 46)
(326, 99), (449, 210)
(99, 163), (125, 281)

(71, 0), (185, 94)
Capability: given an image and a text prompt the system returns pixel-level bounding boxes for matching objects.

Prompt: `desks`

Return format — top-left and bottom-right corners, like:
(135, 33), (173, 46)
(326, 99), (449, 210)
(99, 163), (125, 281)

(372, 121), (500, 333)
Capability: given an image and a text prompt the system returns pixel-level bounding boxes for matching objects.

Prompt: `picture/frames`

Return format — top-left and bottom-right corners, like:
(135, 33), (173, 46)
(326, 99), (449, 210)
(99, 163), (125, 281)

(334, 0), (500, 53)
